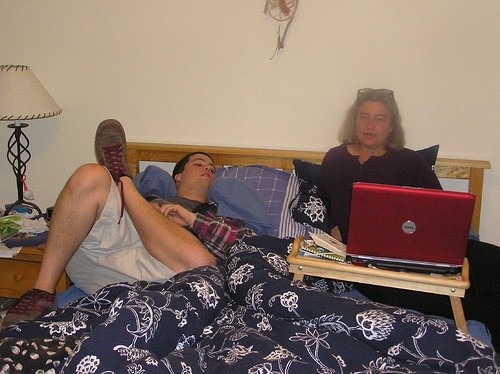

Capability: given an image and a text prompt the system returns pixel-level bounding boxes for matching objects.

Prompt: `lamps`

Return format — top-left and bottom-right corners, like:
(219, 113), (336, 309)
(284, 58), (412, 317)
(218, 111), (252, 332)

(0, 65), (61, 218)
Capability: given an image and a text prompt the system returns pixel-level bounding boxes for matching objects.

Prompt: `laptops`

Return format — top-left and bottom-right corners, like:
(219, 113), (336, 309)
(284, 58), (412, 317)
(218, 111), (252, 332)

(345, 182), (476, 276)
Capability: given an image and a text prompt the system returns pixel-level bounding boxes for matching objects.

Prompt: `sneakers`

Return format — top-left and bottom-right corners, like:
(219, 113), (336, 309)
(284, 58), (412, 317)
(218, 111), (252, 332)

(0, 289), (57, 329)
(95, 119), (133, 224)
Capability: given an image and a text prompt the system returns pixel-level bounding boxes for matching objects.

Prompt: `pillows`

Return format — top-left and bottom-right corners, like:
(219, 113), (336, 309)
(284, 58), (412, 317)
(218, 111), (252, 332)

(214, 145), (438, 237)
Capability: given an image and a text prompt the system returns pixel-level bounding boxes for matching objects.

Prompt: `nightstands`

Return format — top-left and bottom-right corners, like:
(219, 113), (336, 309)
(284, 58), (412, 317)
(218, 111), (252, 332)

(0, 245), (68, 309)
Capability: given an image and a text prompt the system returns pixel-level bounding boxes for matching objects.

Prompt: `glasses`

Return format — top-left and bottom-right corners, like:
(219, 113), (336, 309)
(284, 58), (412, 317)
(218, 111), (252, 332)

(357, 88), (394, 101)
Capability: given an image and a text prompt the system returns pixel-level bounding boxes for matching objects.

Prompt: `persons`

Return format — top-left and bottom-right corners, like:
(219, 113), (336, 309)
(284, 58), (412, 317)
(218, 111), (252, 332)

(322, 87), (443, 243)
(1, 119), (257, 328)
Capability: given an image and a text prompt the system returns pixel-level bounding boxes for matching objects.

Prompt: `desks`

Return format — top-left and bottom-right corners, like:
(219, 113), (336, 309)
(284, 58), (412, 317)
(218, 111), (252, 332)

(287, 235), (470, 337)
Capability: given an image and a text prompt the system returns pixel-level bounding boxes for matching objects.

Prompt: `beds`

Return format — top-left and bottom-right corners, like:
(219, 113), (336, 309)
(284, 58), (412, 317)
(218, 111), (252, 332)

(0, 143), (500, 374)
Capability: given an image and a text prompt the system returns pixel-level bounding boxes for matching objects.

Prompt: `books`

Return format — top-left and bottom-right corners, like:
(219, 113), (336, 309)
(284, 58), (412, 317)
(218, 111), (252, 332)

(298, 233), (347, 262)
